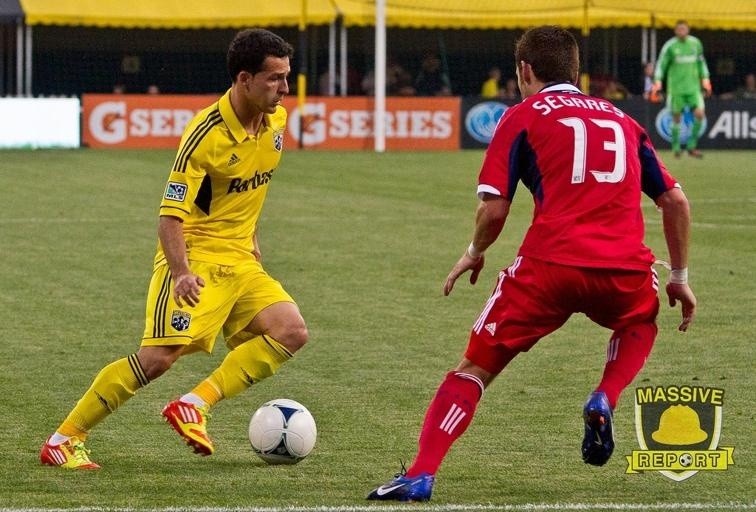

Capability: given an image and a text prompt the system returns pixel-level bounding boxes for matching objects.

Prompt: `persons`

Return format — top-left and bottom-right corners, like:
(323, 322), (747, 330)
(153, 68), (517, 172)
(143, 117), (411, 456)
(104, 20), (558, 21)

(105, 38), (754, 99)
(363, 23), (697, 501)
(650, 20), (714, 158)
(40, 27), (308, 471)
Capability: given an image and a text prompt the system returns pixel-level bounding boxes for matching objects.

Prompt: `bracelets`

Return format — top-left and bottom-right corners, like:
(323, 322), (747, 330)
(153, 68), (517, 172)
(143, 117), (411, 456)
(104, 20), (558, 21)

(465, 240), (484, 259)
(668, 268), (688, 284)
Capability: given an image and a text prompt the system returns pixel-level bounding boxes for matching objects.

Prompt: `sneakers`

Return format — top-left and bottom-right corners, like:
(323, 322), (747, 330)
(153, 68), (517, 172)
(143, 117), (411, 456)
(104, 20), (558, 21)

(160, 398), (215, 458)
(673, 148), (704, 159)
(40, 433), (102, 471)
(364, 471), (436, 504)
(580, 390), (616, 468)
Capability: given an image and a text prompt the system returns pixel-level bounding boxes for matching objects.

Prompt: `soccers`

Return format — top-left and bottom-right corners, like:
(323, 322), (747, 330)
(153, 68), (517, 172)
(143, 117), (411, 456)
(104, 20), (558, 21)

(248, 397), (316, 466)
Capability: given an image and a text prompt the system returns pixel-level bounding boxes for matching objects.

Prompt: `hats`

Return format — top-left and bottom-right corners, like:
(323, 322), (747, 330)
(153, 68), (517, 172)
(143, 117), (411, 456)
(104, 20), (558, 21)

(651, 403), (709, 446)
